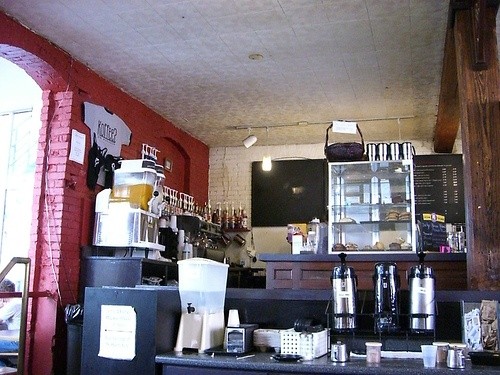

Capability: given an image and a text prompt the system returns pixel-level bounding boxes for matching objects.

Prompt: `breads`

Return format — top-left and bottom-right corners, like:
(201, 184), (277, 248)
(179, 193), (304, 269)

(339, 217), (356, 223)
(333, 238), (411, 252)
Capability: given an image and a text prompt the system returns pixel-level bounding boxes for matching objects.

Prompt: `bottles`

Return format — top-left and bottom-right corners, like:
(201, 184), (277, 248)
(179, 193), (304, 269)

(203, 201), (247, 230)
(193, 244), (198, 257)
(307, 215), (327, 245)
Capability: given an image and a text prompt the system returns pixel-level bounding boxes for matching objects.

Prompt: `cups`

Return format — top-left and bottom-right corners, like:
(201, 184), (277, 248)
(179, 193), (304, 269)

(220, 233), (232, 247)
(365, 342), (382, 365)
(233, 231), (247, 247)
(421, 344), (437, 368)
(170, 215), (193, 260)
(227, 309), (241, 326)
(433, 341), (467, 362)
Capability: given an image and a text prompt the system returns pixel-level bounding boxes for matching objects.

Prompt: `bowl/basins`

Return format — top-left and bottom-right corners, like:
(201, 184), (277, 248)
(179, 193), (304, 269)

(86, 257), (144, 288)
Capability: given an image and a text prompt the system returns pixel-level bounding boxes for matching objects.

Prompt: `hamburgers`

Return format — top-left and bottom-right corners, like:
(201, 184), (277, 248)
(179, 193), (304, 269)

(385, 209), (411, 222)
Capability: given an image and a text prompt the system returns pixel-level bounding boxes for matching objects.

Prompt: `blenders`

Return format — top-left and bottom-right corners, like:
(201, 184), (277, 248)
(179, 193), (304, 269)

(173, 257), (230, 353)
(93, 169), (166, 251)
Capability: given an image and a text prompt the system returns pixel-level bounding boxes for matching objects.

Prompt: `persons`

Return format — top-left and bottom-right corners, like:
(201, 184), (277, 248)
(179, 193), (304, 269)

(0, 279), (22, 375)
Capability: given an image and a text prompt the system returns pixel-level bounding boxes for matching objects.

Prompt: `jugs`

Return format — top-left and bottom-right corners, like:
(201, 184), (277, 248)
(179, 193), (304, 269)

(409, 265), (435, 335)
(330, 341), (348, 362)
(447, 346), (466, 370)
(366, 141), (415, 160)
(332, 265), (359, 331)
(374, 262), (401, 334)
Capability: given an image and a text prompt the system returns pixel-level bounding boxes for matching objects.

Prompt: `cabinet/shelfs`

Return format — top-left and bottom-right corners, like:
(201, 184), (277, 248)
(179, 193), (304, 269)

(78, 247), (265, 305)
(327, 159), (417, 255)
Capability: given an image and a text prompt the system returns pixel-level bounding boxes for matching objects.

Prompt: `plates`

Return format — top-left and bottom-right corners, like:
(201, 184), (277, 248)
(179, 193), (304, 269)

(272, 354), (303, 362)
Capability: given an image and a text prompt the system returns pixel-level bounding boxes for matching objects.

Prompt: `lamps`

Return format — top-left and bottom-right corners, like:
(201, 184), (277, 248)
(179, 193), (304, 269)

(261, 126), (272, 172)
(242, 127), (258, 148)
